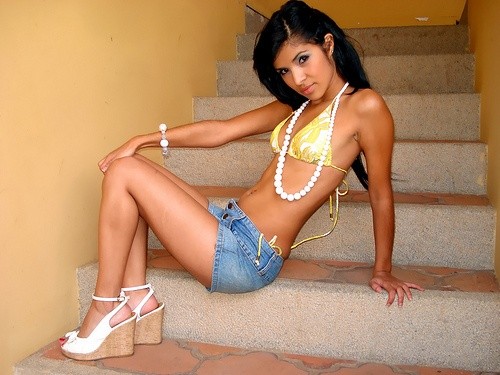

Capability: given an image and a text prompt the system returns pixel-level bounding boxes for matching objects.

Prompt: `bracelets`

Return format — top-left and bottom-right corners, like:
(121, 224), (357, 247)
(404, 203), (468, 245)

(158, 123), (169, 158)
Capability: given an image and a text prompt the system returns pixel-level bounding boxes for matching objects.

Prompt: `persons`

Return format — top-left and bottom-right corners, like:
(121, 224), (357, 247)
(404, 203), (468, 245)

(57, 0), (427, 362)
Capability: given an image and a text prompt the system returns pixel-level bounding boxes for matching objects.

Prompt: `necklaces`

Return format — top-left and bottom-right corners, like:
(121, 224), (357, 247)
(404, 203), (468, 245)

(274, 79), (348, 201)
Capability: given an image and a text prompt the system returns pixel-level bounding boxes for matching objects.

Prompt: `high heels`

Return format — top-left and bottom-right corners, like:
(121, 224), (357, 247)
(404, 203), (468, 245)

(65, 283), (165, 345)
(60, 294), (137, 360)
